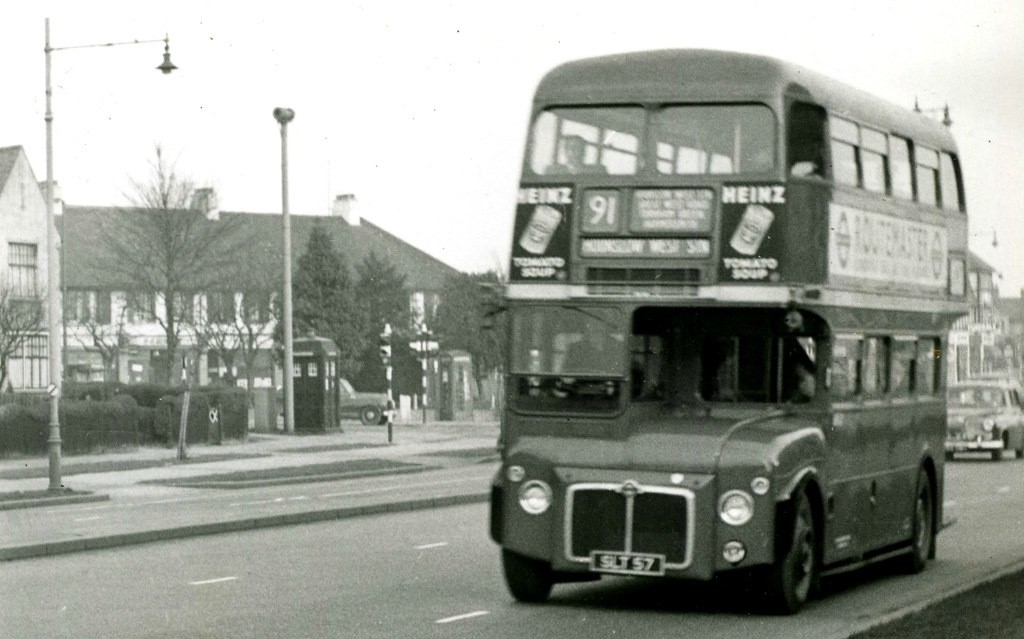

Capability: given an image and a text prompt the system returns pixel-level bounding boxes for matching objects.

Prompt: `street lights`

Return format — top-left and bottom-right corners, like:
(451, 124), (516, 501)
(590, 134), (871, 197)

(36, 15), (181, 493)
(273, 107), (295, 438)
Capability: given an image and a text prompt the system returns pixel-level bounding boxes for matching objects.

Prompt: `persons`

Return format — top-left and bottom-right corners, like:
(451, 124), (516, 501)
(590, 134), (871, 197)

(561, 312), (623, 373)
(545, 135), (607, 175)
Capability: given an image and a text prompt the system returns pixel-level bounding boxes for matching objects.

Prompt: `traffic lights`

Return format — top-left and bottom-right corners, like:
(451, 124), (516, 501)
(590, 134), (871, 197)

(380, 335), (392, 359)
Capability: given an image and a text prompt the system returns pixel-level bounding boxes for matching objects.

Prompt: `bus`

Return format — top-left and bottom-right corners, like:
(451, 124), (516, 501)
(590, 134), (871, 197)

(489, 49), (971, 614)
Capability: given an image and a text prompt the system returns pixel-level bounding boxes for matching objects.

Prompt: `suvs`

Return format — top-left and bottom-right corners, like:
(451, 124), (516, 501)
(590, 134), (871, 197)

(276, 377), (392, 426)
(943, 379), (1024, 461)
